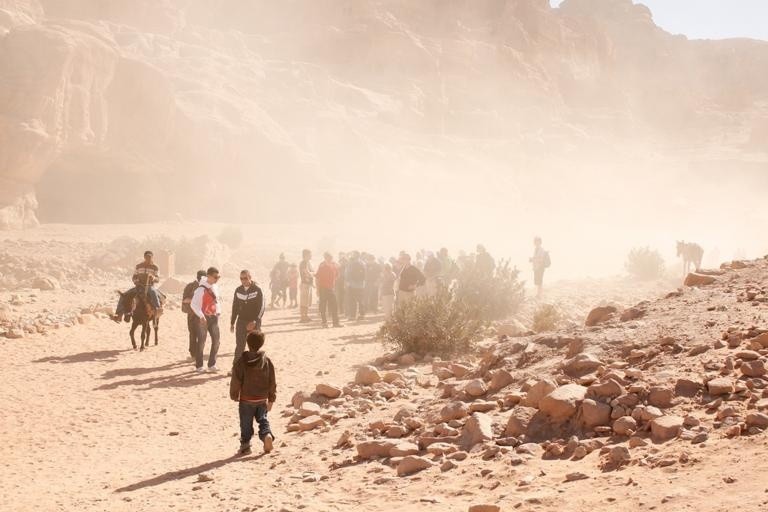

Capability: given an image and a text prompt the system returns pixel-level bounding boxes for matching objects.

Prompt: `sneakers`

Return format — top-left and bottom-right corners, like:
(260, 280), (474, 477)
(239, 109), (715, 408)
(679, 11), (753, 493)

(237, 445), (253, 456)
(196, 366), (207, 374)
(111, 315), (123, 323)
(300, 317), (312, 322)
(263, 433), (274, 453)
(322, 323), (329, 328)
(207, 365), (220, 371)
(333, 323), (345, 328)
(155, 309), (163, 319)
(227, 370), (233, 376)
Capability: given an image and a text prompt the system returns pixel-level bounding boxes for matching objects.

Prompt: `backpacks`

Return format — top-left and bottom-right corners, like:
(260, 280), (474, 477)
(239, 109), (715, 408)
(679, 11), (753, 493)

(542, 250), (551, 268)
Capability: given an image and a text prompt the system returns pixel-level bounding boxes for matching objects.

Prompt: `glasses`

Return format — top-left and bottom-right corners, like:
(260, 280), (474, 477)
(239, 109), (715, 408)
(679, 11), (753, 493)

(211, 275), (221, 279)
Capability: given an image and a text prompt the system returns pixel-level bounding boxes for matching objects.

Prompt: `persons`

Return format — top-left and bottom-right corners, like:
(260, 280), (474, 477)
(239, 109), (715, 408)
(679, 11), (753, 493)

(527, 235), (552, 295)
(229, 331), (277, 457)
(265, 242), (497, 331)
(132, 251), (165, 315)
(182, 269), (208, 361)
(229, 268), (264, 366)
(189, 266), (222, 374)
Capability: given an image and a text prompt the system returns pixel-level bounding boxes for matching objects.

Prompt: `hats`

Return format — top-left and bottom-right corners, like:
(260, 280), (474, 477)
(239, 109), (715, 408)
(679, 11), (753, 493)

(144, 250), (154, 257)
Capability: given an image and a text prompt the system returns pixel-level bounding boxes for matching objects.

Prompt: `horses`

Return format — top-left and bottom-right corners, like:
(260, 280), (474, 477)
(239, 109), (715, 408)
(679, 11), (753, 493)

(675, 238), (704, 276)
(116, 287), (166, 352)
